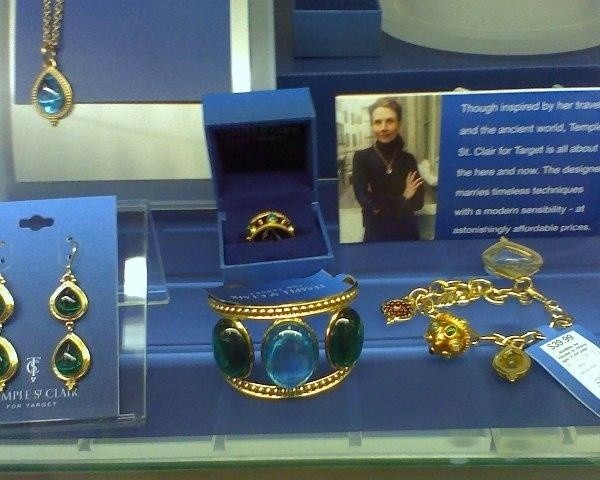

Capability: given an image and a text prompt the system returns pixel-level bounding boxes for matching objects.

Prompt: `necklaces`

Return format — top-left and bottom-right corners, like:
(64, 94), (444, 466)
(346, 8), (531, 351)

(29, 0), (77, 128)
(373, 142), (402, 175)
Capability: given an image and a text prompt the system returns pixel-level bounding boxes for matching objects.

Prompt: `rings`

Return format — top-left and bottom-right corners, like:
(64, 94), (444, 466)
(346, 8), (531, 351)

(240, 209), (297, 242)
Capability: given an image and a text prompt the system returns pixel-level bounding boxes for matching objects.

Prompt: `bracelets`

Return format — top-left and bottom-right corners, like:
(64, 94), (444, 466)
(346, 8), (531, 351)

(200, 271), (367, 404)
(378, 234), (574, 382)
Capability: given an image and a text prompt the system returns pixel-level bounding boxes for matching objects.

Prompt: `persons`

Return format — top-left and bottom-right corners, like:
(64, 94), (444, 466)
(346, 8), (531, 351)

(350, 98), (425, 244)
